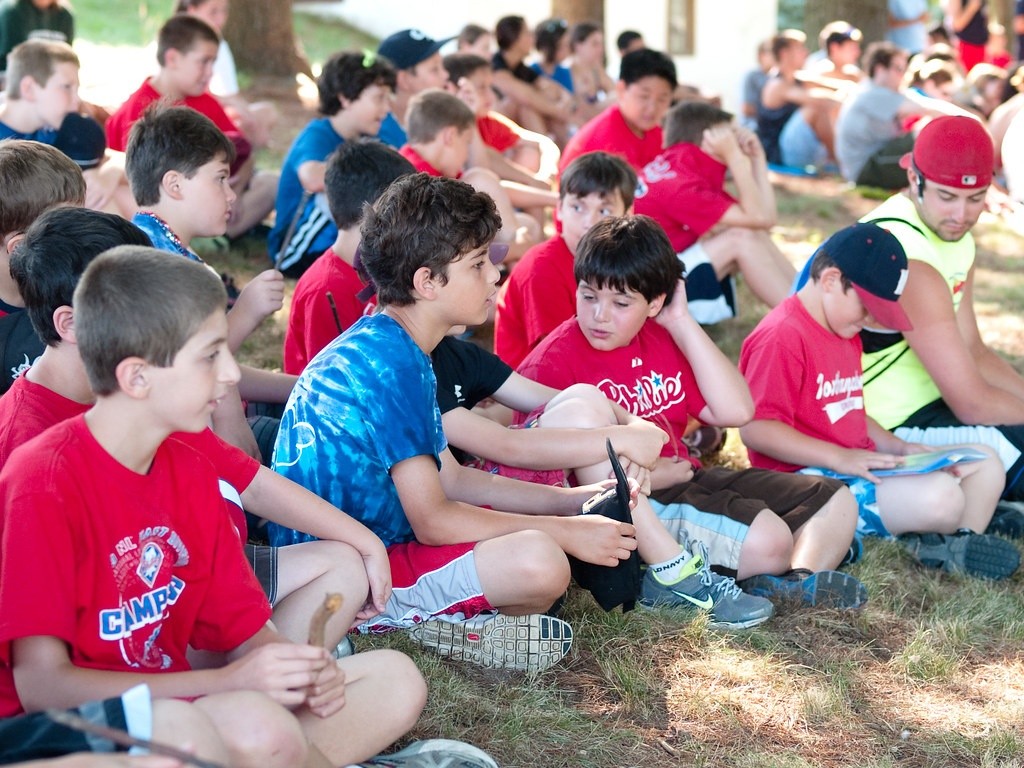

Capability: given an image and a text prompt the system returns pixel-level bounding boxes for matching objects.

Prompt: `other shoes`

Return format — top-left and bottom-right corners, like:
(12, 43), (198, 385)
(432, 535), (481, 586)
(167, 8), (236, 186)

(409, 614), (573, 675)
(898, 528), (1019, 581)
(741, 570), (868, 609)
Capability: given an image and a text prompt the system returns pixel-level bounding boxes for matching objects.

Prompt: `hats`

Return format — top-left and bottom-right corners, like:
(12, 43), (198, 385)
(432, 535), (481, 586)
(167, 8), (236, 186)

(824, 222), (913, 331)
(378, 29), (458, 71)
(900, 116), (994, 189)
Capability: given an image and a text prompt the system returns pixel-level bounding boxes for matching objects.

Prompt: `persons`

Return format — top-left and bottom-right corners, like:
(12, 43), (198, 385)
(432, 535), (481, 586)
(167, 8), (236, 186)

(0, 244), (500, 768)
(267, 1), (871, 665)
(1, 0), (300, 544)
(736, 0), (1024, 585)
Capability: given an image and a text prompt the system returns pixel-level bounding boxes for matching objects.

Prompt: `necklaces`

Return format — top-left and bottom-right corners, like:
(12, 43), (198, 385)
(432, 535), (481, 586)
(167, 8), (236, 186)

(139, 211), (187, 256)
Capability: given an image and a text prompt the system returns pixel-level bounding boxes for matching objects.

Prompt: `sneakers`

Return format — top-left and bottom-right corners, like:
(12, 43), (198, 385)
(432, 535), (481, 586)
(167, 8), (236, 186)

(345, 739), (498, 768)
(637, 540), (772, 630)
